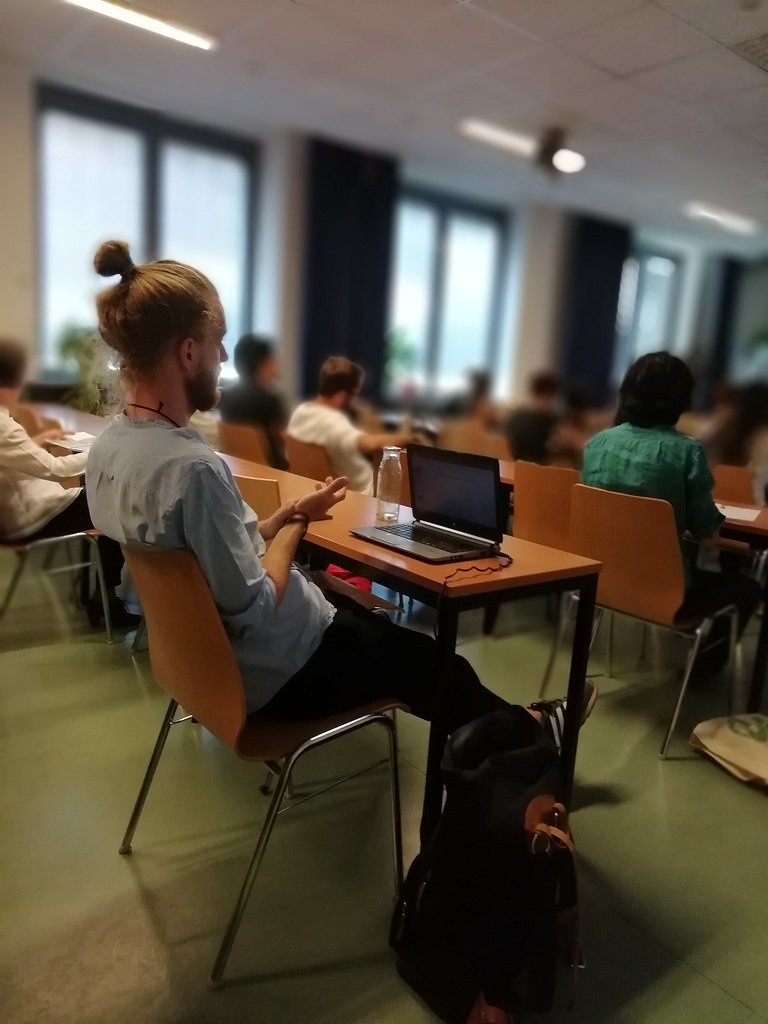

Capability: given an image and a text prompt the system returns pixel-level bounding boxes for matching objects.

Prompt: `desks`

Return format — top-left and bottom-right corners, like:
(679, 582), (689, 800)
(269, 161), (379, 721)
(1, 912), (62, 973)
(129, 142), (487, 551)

(399, 447), (768, 715)
(20, 398), (603, 858)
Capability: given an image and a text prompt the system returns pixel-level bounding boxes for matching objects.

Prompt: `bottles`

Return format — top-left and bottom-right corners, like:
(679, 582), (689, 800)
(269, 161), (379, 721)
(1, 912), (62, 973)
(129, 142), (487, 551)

(377, 446), (403, 522)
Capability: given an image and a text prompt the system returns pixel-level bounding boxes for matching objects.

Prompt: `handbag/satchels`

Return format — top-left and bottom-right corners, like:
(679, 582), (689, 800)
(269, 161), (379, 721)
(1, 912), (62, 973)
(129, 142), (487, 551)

(690, 713), (768, 788)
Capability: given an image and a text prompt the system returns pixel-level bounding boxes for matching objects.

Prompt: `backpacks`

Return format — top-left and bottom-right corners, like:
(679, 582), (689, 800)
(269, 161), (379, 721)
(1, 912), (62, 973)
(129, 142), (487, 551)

(389, 705), (586, 1024)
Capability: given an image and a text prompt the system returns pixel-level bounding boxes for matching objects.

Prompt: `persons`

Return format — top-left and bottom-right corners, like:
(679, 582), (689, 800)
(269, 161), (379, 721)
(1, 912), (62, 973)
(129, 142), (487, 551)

(219, 334), (294, 471)
(283, 356), (430, 497)
(462, 369), (767, 506)
(1, 342), (142, 633)
(94, 238), (598, 758)
(580, 353), (758, 691)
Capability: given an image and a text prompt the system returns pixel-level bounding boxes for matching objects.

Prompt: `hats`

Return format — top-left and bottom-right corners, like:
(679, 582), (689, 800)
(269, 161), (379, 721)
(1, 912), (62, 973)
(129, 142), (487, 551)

(311, 563), (400, 611)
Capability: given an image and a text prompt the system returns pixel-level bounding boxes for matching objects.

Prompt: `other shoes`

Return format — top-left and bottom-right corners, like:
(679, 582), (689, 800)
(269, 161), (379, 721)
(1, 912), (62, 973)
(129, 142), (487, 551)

(678, 668), (719, 689)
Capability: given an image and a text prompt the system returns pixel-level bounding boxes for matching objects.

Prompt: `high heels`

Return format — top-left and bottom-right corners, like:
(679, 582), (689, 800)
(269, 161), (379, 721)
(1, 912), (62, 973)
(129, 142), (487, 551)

(87, 594), (141, 629)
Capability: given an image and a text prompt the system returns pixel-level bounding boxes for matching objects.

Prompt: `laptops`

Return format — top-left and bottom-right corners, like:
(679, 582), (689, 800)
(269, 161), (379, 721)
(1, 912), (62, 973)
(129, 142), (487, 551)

(349, 442), (502, 566)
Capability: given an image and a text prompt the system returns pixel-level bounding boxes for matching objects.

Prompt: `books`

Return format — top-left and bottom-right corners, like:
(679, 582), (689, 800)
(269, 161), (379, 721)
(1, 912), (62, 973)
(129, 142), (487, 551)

(44, 432), (97, 452)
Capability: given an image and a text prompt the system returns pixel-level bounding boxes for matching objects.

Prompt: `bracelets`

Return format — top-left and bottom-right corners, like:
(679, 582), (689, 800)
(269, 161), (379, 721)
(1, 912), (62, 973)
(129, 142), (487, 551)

(285, 512), (310, 539)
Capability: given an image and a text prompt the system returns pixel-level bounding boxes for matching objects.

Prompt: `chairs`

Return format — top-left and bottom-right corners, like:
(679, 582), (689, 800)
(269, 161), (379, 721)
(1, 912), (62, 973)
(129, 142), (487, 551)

(279, 429), (338, 485)
(118, 537), (407, 981)
(710, 460), (768, 642)
(540, 482), (740, 758)
(512, 459), (591, 644)
(0, 528), (115, 645)
(215, 422), (270, 467)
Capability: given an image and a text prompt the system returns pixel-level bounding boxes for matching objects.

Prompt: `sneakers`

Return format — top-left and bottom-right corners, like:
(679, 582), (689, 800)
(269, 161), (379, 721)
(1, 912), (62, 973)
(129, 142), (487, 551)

(529, 678), (598, 757)
